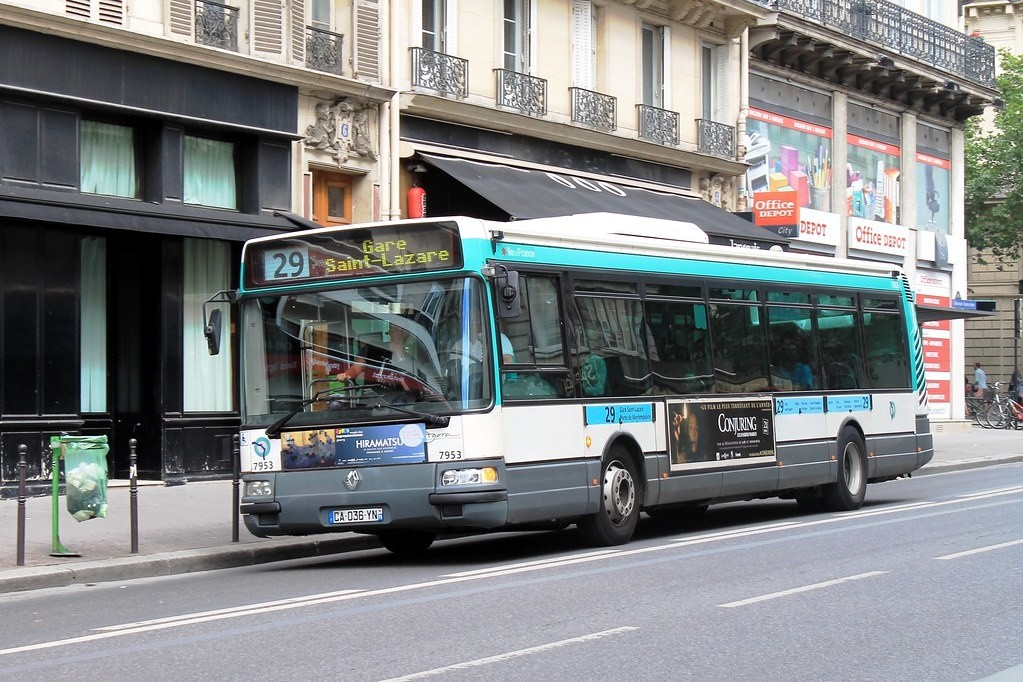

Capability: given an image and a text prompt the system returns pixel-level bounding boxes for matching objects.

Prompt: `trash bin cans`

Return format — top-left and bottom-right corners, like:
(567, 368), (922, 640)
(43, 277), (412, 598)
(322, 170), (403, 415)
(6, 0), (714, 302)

(60, 435), (109, 522)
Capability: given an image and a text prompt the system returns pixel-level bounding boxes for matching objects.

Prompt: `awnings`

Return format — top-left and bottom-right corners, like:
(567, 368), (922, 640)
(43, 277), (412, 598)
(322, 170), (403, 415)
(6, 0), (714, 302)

(400, 143), (791, 253)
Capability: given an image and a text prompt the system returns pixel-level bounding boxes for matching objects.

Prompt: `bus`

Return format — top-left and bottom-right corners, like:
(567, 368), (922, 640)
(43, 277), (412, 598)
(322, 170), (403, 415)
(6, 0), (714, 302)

(202, 212), (932, 553)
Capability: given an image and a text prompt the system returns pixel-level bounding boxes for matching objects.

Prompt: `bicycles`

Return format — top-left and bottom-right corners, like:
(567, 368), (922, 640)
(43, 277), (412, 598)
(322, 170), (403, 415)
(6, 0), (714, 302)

(964, 382), (1023, 430)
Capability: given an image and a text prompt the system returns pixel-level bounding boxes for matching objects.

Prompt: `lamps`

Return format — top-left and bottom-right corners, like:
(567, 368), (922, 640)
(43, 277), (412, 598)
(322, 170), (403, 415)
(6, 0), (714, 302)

(940, 79), (961, 90)
(874, 53), (893, 68)
(991, 98), (1004, 107)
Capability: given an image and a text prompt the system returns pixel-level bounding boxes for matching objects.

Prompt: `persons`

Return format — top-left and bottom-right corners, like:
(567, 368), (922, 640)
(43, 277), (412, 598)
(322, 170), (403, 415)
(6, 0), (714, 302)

(965, 363), (987, 414)
(337, 315), (424, 406)
(774, 344), (813, 391)
(1008, 363), (1023, 407)
(672, 411), (709, 464)
(444, 304), (518, 385)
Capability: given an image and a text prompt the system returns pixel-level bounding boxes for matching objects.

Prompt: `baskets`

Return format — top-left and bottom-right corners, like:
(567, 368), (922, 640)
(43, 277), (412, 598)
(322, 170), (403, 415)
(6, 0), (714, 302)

(983, 388), (996, 400)
